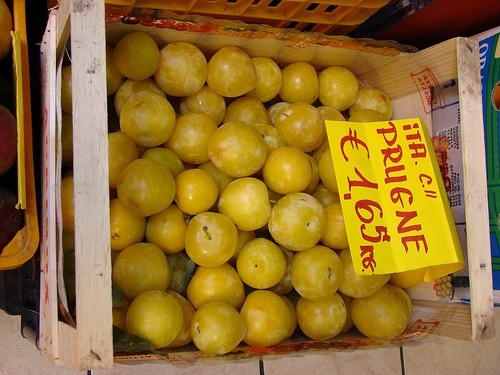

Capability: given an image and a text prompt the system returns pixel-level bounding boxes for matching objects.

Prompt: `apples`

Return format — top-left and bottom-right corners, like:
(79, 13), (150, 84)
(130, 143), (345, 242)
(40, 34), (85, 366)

(0, 0), (22, 255)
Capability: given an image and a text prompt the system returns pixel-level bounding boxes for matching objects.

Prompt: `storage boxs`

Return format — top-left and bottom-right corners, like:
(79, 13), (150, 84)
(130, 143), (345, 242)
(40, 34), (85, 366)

(0, 1), (41, 270)
(404, 25), (499, 306)
(22, 0), (495, 368)
(105, 0), (390, 37)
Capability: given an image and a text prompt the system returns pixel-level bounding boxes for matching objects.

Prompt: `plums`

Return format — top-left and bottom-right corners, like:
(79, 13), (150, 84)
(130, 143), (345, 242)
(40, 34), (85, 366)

(60, 30), (427, 356)
